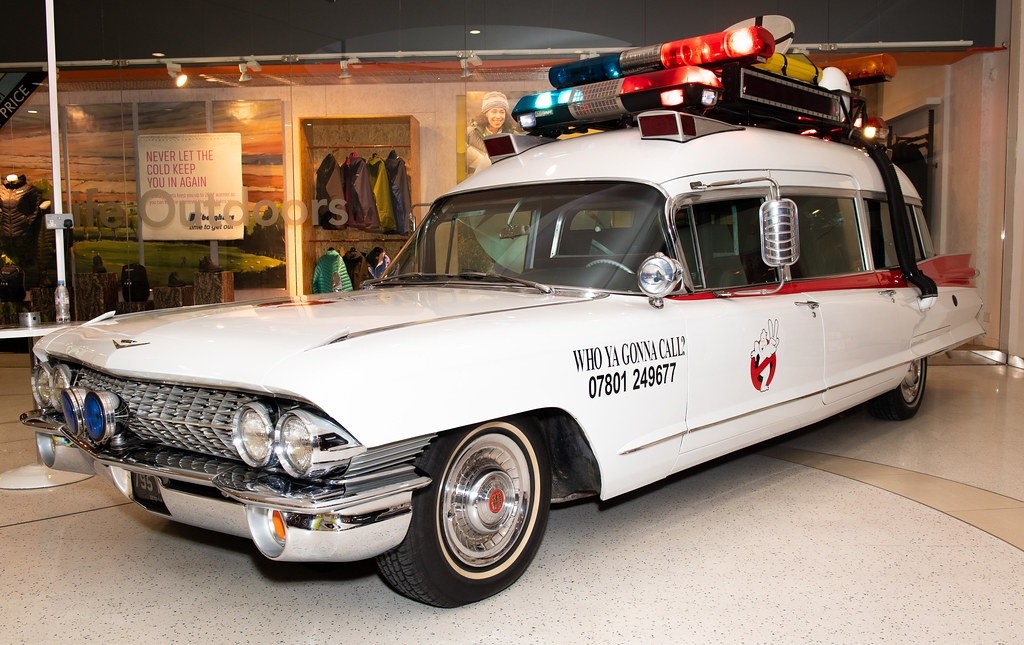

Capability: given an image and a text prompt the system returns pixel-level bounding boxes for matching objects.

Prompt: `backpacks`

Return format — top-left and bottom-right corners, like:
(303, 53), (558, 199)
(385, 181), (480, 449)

(121, 263), (151, 302)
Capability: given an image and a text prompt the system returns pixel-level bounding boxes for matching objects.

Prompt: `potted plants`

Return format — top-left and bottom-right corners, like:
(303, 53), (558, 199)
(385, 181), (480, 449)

(18, 308), (42, 326)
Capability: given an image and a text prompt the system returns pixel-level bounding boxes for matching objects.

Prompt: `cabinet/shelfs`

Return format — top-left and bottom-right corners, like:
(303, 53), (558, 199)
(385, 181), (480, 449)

(297, 113), (420, 296)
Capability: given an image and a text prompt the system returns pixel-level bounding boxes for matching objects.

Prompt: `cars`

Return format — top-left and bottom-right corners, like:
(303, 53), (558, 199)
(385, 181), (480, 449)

(18, 26), (988, 611)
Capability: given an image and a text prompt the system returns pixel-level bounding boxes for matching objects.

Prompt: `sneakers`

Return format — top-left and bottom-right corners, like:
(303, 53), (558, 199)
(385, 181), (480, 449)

(199, 254), (223, 273)
(167, 271), (185, 287)
(92, 255), (107, 274)
(38, 270), (52, 288)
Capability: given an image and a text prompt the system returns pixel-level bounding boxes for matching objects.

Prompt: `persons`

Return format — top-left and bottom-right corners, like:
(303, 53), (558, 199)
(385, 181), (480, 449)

(468, 91), (519, 169)
(0, 174), (56, 278)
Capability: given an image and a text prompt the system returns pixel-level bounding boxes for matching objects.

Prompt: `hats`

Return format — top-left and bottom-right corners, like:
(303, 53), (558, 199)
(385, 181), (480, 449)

(480, 92), (509, 114)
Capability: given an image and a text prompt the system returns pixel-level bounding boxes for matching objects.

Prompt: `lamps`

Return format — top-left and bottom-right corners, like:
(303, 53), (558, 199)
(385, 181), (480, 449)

(167, 63), (191, 86)
(238, 60), (262, 83)
(340, 57), (362, 78)
(461, 55), (484, 78)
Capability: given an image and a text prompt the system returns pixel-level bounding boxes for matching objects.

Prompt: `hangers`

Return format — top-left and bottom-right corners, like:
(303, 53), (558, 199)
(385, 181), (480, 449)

(324, 239), (381, 250)
(324, 145), (397, 156)
